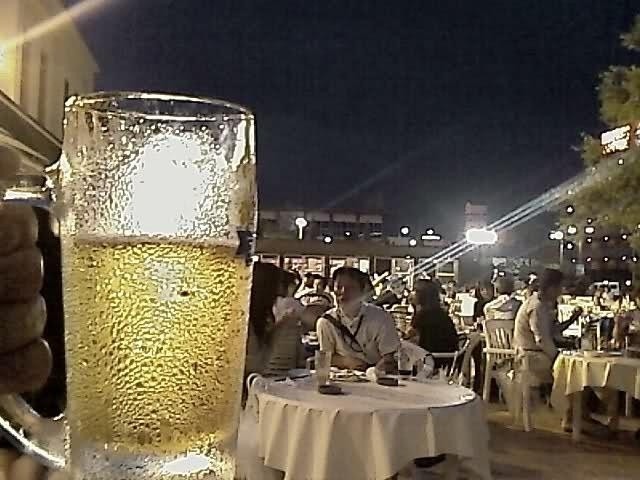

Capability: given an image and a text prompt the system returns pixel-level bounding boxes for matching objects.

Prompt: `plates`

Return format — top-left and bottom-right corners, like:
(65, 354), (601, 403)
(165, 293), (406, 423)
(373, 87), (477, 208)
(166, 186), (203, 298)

(584, 350), (605, 358)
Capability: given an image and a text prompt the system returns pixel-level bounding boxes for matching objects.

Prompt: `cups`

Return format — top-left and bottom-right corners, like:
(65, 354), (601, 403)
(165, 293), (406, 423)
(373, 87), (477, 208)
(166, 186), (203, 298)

(305, 350), (333, 387)
(0, 91), (257, 480)
(397, 345), (413, 381)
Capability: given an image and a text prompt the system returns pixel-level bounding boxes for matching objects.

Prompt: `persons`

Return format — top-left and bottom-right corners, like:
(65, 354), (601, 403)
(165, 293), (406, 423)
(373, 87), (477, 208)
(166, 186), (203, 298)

(471, 269), (639, 437)
(1, 146), (68, 480)
(370, 273), (459, 377)
(244, 264), (400, 377)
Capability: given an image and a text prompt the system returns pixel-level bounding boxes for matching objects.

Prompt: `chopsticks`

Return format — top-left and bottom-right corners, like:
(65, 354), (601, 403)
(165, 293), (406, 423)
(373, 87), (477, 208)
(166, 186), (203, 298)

(273, 374), (310, 381)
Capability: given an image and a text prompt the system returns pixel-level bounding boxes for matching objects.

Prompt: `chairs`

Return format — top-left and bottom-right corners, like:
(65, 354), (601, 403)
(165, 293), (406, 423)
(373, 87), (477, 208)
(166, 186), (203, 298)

(306, 294), (549, 433)
